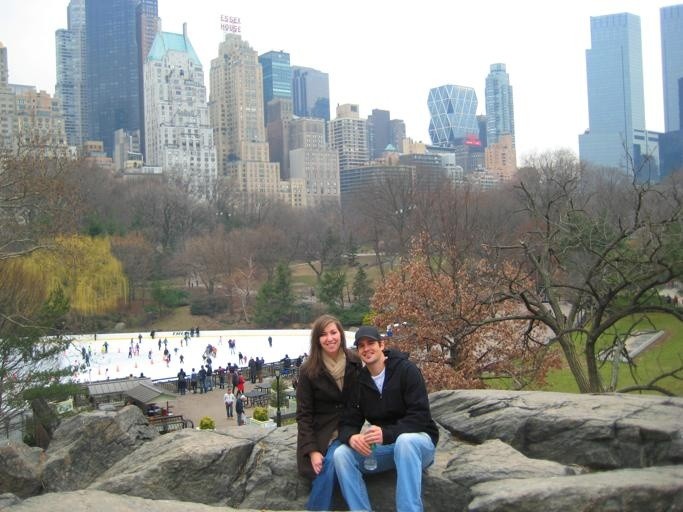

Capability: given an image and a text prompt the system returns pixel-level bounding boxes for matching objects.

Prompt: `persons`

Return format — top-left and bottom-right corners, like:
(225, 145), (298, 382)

(128, 374), (136, 378)
(333, 327), (439, 512)
(173, 339), (264, 393)
(301, 352), (308, 359)
(296, 355), (302, 367)
(267, 336), (272, 346)
(278, 354), (291, 370)
(235, 394), (246, 426)
(223, 389), (235, 419)
(138, 372), (146, 378)
(295, 315), (358, 511)
(76, 328), (200, 371)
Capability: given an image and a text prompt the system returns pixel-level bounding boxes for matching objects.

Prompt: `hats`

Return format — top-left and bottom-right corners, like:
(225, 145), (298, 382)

(354, 327), (380, 345)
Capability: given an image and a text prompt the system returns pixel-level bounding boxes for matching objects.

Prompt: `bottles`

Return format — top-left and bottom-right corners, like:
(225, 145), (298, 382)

(361, 420), (379, 472)
